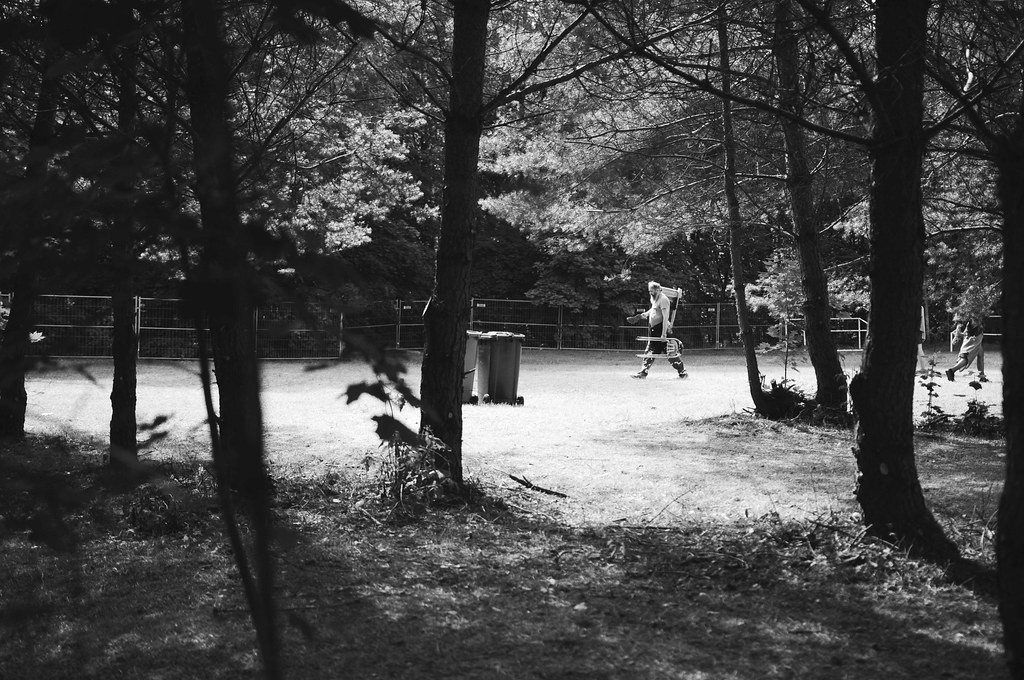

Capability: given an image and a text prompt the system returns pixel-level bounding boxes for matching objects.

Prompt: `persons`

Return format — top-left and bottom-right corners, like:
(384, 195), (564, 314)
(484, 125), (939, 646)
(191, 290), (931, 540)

(944, 284), (990, 382)
(918, 305), (926, 372)
(626, 280), (689, 381)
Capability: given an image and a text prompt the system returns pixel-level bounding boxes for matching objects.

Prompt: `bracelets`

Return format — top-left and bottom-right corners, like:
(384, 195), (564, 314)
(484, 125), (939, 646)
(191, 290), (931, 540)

(641, 313), (648, 320)
(662, 334), (666, 337)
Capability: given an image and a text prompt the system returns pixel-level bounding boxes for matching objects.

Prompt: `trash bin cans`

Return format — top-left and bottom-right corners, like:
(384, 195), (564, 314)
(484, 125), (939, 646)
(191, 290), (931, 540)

(478, 331), (526, 404)
(462, 329), (482, 405)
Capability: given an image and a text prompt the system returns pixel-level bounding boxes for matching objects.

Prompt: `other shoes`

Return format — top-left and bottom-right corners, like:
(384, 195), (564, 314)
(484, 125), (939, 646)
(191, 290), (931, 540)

(915, 369), (928, 376)
(979, 377), (989, 383)
(946, 368), (954, 382)
(630, 371), (647, 380)
(679, 369), (689, 379)
